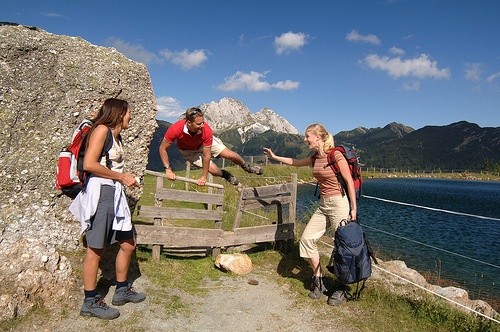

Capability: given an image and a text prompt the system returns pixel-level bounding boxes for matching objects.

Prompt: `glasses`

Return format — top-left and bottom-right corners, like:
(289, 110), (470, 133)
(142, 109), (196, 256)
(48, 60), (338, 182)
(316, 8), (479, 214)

(189, 108), (202, 117)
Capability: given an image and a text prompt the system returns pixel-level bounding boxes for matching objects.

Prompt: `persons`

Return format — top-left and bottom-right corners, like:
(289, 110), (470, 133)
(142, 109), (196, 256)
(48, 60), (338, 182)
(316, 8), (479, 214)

(263, 122), (356, 306)
(69, 98), (146, 320)
(159, 107), (263, 186)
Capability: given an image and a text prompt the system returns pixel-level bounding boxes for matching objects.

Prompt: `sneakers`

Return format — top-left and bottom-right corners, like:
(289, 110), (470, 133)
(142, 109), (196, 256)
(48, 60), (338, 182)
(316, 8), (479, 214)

(308, 275), (332, 298)
(240, 162), (264, 175)
(328, 289), (347, 306)
(112, 282), (146, 306)
(221, 169), (239, 186)
(80, 291), (120, 319)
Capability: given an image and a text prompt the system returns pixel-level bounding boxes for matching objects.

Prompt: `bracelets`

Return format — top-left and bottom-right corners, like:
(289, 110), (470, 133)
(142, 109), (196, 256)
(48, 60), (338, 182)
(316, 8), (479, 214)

(164, 165), (171, 169)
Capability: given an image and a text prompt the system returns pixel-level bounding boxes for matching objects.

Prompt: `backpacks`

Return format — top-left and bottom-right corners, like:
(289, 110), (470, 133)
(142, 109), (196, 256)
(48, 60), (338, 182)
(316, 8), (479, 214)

(326, 218), (378, 284)
(313, 145), (365, 201)
(54, 117), (123, 200)
